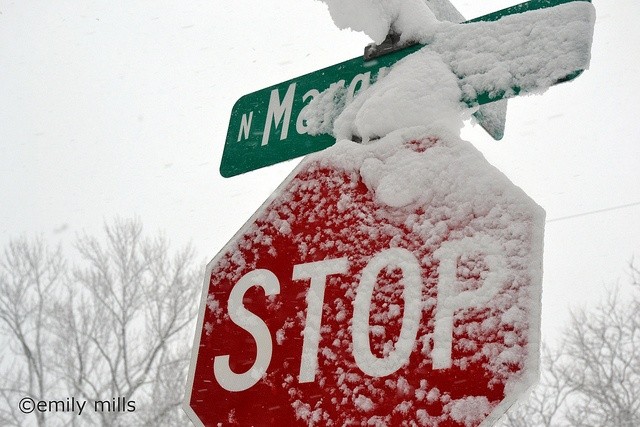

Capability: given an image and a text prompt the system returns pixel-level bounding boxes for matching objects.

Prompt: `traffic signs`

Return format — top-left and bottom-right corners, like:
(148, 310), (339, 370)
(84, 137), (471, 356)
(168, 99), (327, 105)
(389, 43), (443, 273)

(217, 0), (594, 179)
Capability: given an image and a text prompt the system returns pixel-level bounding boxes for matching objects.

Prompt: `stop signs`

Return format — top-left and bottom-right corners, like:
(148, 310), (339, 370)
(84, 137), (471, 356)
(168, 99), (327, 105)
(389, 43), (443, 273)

(187, 135), (544, 427)
